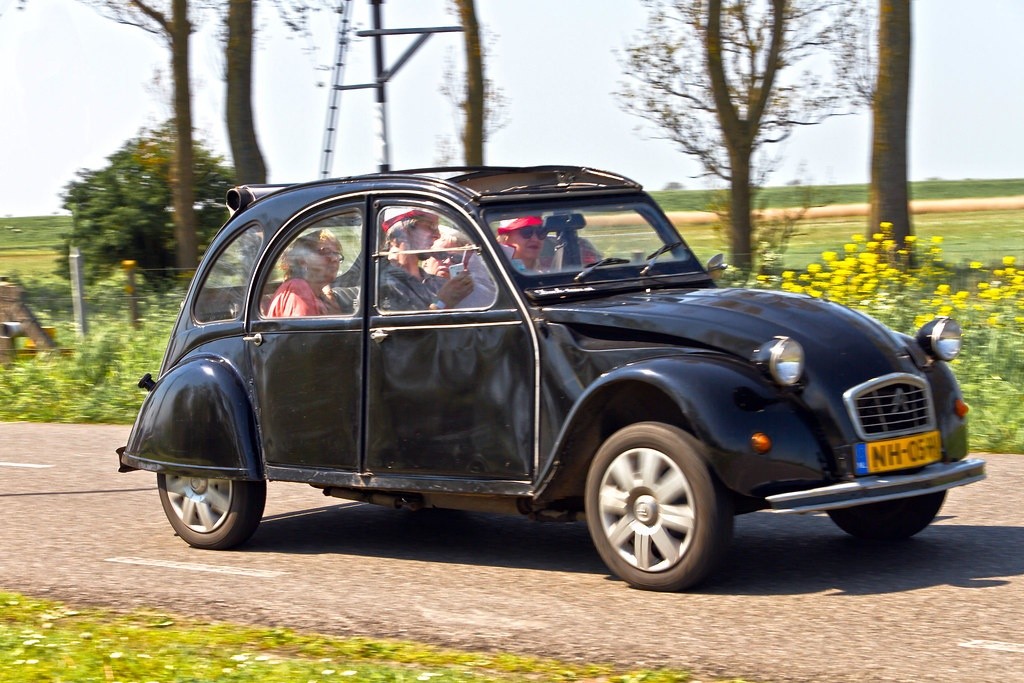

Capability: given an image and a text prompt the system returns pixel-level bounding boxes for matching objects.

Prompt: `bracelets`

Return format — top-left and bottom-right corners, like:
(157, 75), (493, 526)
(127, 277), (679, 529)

(436, 299), (446, 309)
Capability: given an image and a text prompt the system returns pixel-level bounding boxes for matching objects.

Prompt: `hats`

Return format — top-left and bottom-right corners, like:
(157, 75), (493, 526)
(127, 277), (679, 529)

(498, 216), (544, 236)
(382, 208), (438, 232)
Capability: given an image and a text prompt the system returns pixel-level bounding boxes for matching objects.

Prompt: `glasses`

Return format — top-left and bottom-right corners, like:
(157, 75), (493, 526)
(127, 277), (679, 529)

(514, 225), (548, 241)
(307, 247), (344, 265)
(417, 216), (440, 231)
(431, 251), (463, 265)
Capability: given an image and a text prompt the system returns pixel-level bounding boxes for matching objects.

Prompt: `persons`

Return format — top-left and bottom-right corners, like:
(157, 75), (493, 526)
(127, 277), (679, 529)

(267, 229), (343, 318)
(378, 207), (475, 313)
(496, 216), (548, 273)
(421, 224), (474, 280)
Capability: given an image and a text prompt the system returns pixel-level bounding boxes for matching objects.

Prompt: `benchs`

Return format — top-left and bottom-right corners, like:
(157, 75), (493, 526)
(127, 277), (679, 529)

(226, 284), (361, 315)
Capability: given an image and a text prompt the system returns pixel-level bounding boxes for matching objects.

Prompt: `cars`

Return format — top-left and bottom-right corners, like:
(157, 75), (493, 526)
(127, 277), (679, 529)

(112, 163), (986, 592)
